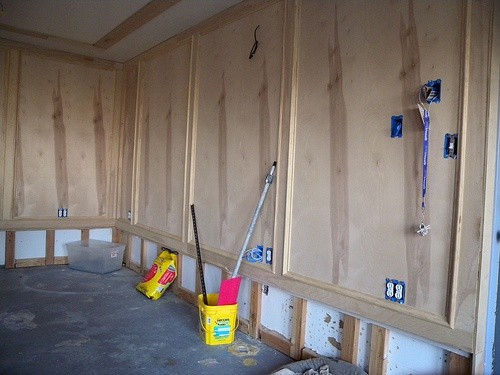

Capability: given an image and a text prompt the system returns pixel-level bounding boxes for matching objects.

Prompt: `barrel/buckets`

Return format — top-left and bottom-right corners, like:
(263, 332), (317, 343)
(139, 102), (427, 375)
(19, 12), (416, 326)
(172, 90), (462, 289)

(197, 292), (240, 345)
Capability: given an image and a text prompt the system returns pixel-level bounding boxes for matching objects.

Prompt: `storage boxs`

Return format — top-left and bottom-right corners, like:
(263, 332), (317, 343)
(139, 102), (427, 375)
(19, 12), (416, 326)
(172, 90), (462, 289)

(66, 239), (126, 275)
